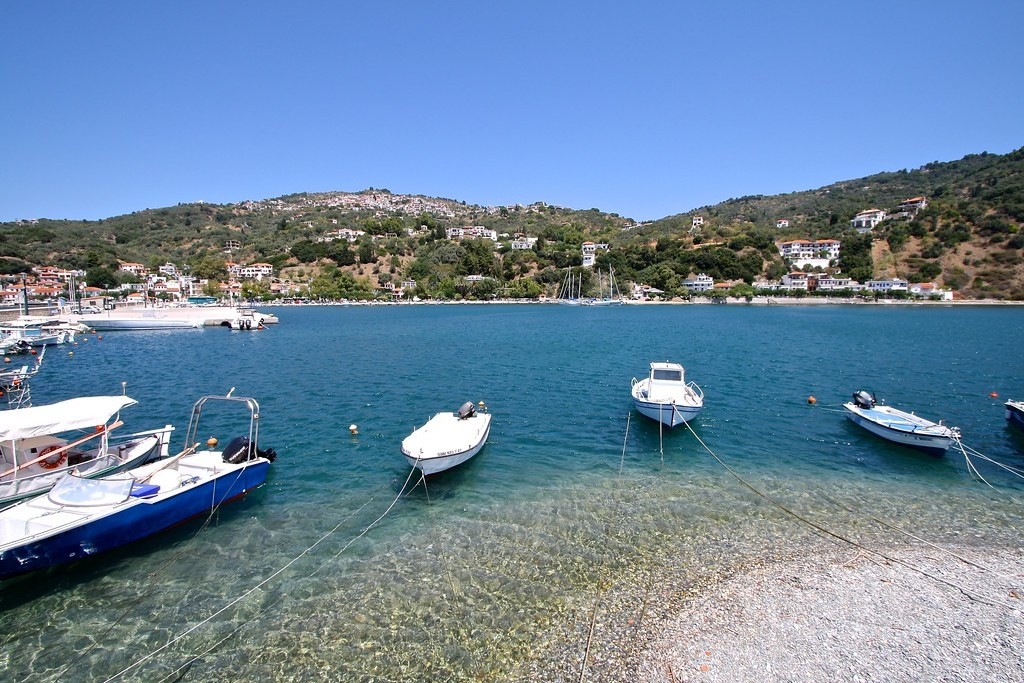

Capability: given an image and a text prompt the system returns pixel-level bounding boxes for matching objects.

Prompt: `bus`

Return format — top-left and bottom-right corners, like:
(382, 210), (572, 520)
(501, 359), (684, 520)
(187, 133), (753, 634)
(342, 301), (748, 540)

(187, 297), (217, 303)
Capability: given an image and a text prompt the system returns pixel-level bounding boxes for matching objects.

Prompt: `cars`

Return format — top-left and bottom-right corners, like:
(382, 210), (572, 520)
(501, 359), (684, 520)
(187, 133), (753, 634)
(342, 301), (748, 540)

(72, 306), (102, 314)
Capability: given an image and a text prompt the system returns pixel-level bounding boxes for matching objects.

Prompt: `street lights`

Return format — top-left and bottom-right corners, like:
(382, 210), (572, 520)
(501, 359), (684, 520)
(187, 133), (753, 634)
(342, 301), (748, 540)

(21, 273), (29, 315)
(102, 283), (110, 316)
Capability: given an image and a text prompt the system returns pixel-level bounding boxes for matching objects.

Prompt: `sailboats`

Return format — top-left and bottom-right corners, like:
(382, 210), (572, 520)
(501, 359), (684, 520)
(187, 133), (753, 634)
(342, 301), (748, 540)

(551, 263), (623, 308)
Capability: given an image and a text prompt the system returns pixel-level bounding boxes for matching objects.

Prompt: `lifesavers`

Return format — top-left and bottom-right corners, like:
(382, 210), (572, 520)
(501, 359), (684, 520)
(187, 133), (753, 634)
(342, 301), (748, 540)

(38, 446), (67, 469)
(96, 425), (105, 431)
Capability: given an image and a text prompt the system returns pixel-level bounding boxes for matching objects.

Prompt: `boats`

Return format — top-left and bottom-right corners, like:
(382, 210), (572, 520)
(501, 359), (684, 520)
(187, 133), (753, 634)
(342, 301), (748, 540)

(1005, 400), (1024, 424)
(0, 327), (58, 355)
(401, 400), (493, 478)
(1, 397), (160, 502)
(843, 389), (958, 451)
(231, 312), (259, 330)
(0, 393), (273, 584)
(631, 359), (703, 426)
